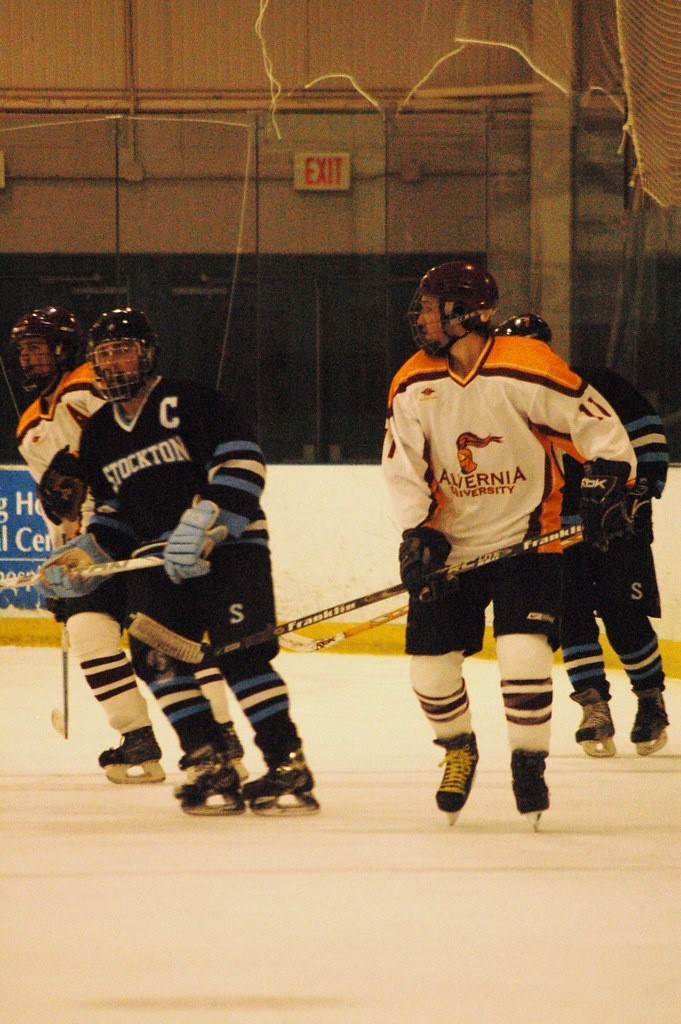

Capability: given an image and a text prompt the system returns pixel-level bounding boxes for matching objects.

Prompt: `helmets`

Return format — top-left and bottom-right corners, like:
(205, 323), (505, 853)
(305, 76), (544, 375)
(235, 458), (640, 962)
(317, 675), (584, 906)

(406, 261), (499, 358)
(494, 312), (552, 348)
(5, 306), (83, 396)
(84, 307), (157, 403)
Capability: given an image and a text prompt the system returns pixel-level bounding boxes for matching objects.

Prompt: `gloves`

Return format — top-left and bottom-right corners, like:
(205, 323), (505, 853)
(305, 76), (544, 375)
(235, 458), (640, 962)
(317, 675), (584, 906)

(36, 533), (117, 599)
(164, 493), (229, 584)
(581, 457), (631, 554)
(619, 476), (655, 546)
(399, 526), (460, 605)
(40, 445), (88, 525)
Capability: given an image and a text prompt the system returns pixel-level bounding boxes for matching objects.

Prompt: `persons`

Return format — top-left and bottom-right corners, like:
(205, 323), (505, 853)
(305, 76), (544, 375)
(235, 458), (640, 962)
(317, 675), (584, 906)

(33, 304), (322, 817)
(8, 306), (251, 785)
(491, 313), (670, 757)
(381, 258), (639, 833)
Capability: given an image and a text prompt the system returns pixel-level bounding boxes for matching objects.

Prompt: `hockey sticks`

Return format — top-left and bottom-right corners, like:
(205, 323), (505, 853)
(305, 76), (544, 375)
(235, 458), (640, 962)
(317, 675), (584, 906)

(127, 522), (587, 665)
(1, 538), (211, 593)
(275, 532), (583, 653)
(50, 532), (70, 740)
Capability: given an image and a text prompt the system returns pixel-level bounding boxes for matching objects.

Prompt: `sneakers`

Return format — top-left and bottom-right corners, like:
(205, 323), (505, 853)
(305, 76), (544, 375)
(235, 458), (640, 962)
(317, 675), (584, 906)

(218, 721), (248, 781)
(432, 730), (479, 826)
(241, 737), (321, 817)
(174, 741), (247, 817)
(630, 688), (669, 756)
(511, 748), (550, 833)
(99, 727), (166, 784)
(570, 687), (617, 756)
